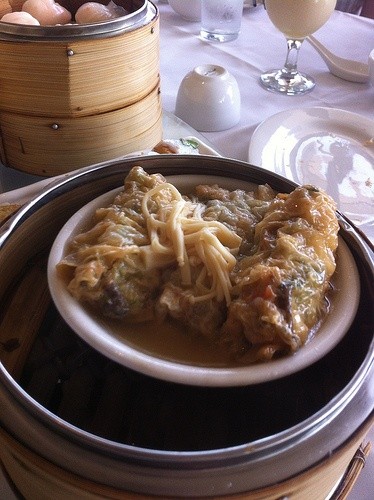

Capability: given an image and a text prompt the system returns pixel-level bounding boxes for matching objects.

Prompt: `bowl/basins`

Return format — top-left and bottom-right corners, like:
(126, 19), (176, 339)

(168, 0), (201, 21)
(174, 64), (241, 132)
(48, 175), (360, 386)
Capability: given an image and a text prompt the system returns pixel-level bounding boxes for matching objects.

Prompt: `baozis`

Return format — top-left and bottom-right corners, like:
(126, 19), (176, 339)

(0, 0), (122, 26)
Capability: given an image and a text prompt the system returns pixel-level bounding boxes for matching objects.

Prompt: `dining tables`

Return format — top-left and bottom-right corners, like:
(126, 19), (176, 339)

(0, 0), (373, 500)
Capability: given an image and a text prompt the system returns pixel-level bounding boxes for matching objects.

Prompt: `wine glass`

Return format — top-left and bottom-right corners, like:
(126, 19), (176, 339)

(260, 0), (337, 96)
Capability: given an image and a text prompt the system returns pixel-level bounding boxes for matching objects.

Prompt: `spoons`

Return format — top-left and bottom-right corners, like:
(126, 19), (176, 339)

(306, 35), (370, 82)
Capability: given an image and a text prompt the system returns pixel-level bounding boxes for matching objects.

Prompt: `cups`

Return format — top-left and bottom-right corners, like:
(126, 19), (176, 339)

(200, 0), (243, 42)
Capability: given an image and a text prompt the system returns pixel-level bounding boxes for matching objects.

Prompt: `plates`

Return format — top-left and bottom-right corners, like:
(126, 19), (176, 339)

(0, 136), (223, 228)
(248, 106), (374, 226)
